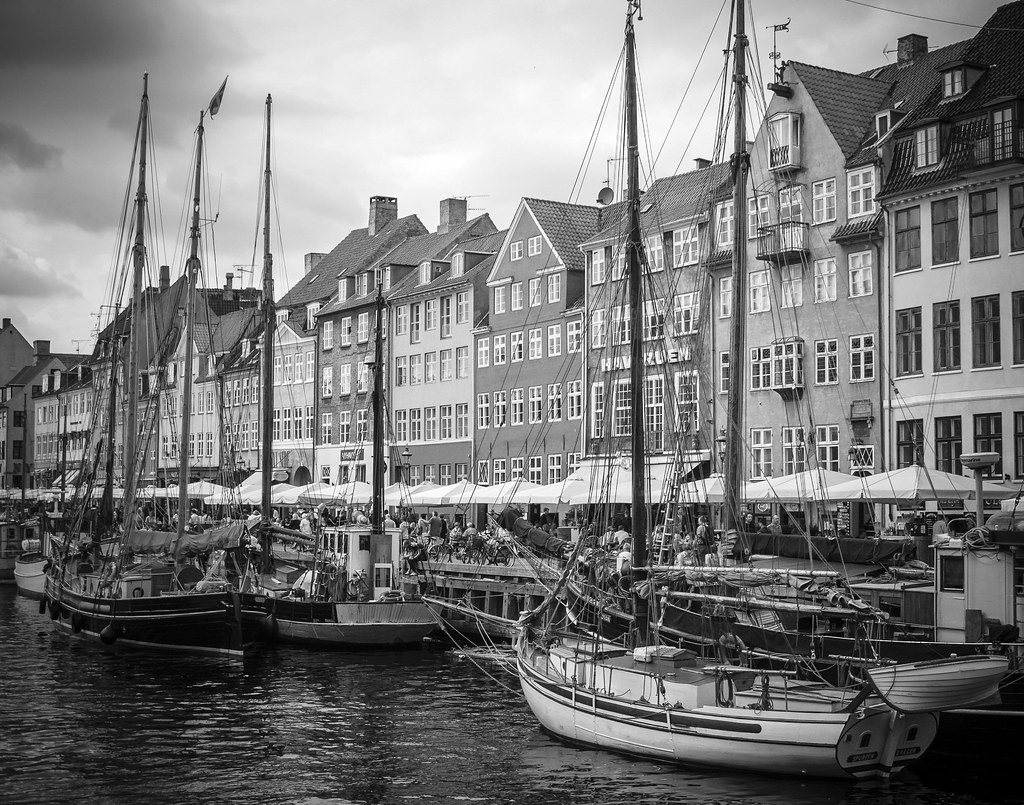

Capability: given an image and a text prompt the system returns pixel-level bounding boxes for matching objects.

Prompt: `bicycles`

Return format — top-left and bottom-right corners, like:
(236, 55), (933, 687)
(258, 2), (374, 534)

(420, 531), (516, 568)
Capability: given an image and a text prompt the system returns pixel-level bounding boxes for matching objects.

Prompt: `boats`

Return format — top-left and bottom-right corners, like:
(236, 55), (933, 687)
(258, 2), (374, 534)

(867, 652), (1011, 712)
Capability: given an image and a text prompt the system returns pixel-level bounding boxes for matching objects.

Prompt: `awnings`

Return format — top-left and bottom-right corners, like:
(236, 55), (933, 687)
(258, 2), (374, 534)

(563, 461), (700, 483)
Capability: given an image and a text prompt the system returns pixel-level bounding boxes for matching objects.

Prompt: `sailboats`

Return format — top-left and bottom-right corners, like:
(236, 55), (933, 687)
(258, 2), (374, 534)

(0, 0), (1024, 782)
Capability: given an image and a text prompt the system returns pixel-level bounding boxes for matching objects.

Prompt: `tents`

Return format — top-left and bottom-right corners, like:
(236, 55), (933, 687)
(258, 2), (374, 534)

(0, 469), (1024, 506)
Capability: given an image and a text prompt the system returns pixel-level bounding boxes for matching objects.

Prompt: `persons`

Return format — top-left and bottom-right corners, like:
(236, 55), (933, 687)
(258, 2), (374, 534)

(39, 502), (786, 570)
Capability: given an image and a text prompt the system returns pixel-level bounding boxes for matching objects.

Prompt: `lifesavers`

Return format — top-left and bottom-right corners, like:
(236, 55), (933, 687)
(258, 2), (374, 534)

(716, 632), (749, 668)
(380, 589), (408, 601)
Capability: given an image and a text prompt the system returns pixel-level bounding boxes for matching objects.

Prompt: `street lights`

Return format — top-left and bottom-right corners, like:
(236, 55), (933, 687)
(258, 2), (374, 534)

(236, 455), (245, 484)
(714, 425), (727, 529)
(402, 443), (413, 517)
